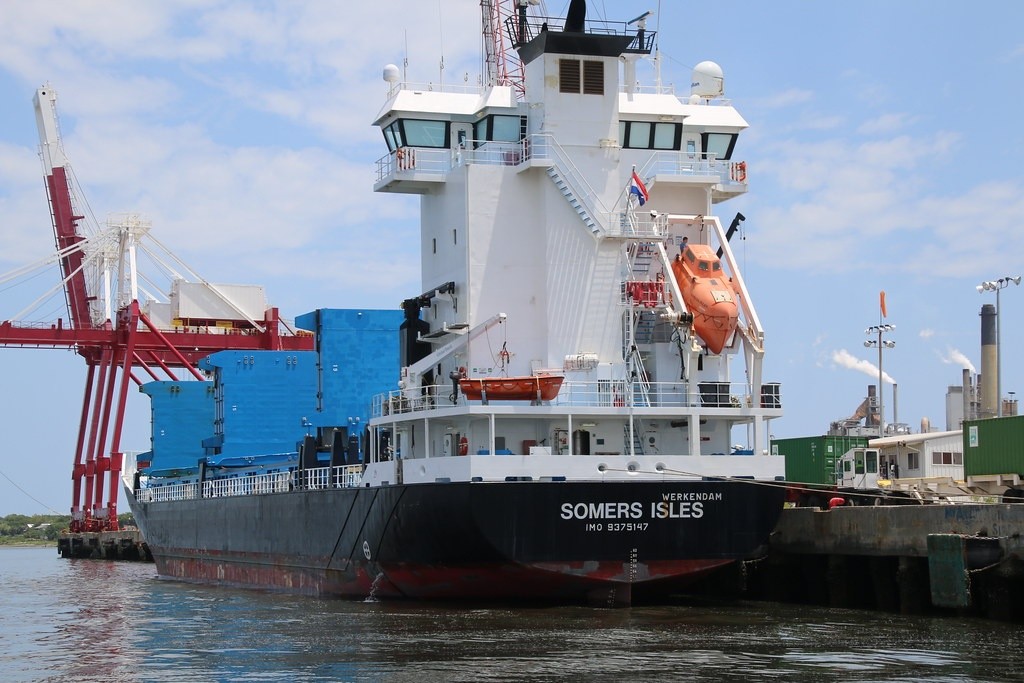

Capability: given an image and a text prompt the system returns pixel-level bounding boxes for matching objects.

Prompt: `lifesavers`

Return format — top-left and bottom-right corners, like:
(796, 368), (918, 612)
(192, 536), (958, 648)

(729, 162), (747, 182)
(458, 437), (468, 457)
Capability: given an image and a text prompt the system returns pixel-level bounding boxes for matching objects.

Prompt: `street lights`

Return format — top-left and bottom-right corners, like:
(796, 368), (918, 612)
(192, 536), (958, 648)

(975, 276), (1021, 418)
(865, 325), (897, 435)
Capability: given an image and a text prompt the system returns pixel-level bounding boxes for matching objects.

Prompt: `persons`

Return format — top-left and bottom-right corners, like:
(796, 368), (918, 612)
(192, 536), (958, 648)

(679, 236), (689, 254)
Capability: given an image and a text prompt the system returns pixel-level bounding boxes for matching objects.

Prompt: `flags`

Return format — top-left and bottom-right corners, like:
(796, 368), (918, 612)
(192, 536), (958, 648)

(880, 292), (887, 317)
(631, 174), (648, 205)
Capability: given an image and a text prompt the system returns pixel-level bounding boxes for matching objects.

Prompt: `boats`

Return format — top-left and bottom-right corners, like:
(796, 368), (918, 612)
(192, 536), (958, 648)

(669, 243), (739, 355)
(459, 376), (565, 402)
(122, 0), (799, 610)
